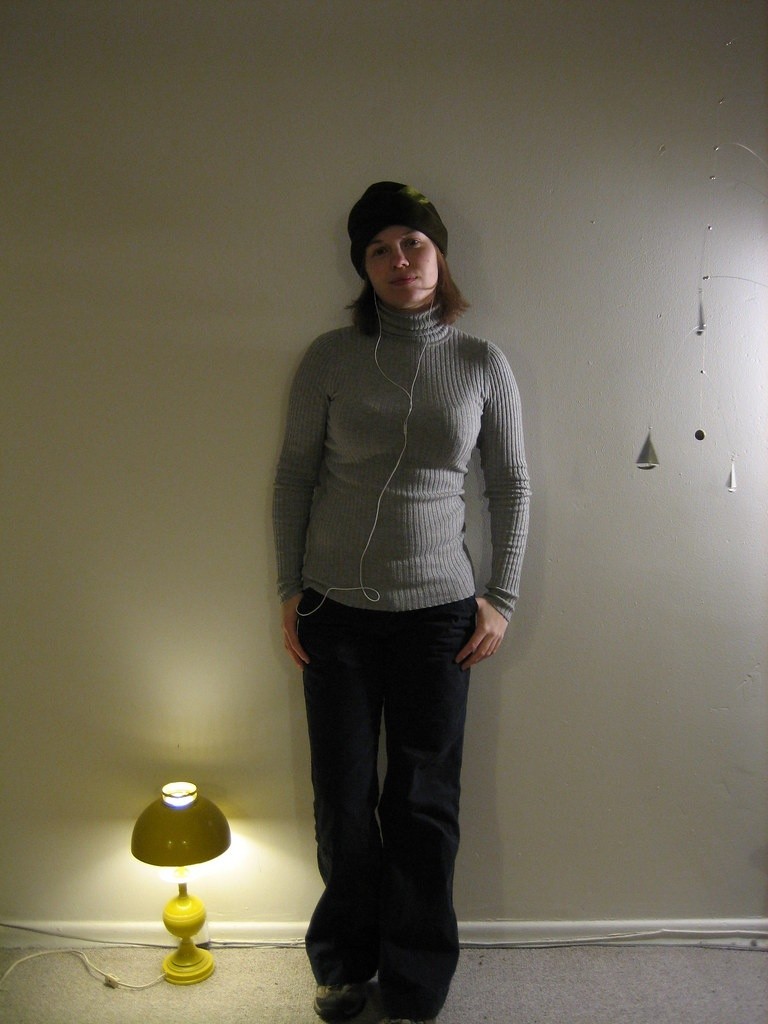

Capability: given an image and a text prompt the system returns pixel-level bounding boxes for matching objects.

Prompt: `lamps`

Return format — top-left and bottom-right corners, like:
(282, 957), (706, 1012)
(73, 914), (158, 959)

(131, 781), (232, 986)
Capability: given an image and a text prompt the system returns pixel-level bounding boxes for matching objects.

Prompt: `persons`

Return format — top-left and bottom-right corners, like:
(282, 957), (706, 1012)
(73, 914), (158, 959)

(270, 180), (532, 1024)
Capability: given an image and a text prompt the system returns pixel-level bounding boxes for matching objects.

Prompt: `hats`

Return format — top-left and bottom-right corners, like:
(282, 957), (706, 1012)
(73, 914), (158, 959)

(348, 181), (448, 277)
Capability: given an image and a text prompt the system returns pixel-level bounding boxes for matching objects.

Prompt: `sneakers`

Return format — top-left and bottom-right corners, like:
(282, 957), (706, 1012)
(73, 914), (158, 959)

(313, 982), (365, 1021)
(383, 1012), (437, 1024)
